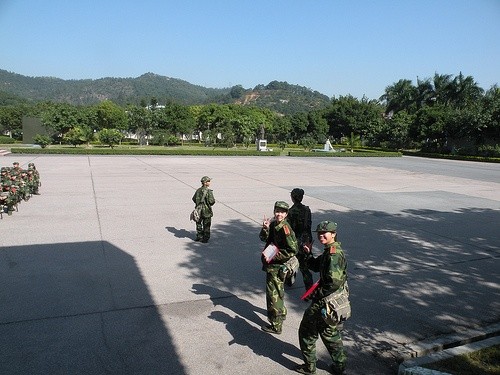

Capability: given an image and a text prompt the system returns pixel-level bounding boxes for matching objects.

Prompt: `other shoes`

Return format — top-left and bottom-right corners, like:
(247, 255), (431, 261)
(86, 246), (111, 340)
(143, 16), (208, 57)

(333, 364), (347, 375)
(195, 237), (209, 243)
(262, 325), (282, 334)
(295, 365), (317, 375)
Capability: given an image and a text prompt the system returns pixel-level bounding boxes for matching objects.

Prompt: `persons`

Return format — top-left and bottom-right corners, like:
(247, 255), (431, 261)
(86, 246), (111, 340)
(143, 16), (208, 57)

(0, 162), (42, 216)
(296, 220), (352, 375)
(285, 188), (314, 301)
(192, 176), (215, 243)
(259, 201), (299, 334)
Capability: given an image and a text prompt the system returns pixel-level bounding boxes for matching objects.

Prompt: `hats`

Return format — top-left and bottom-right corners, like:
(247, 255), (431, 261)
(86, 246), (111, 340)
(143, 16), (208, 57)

(288, 188), (305, 201)
(201, 176), (214, 183)
(274, 201), (289, 211)
(312, 221), (338, 233)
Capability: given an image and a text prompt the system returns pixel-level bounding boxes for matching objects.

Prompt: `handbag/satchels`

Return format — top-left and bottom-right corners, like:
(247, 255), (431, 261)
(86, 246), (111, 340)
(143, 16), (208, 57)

(283, 256), (300, 278)
(319, 282), (352, 329)
(190, 204), (202, 223)
